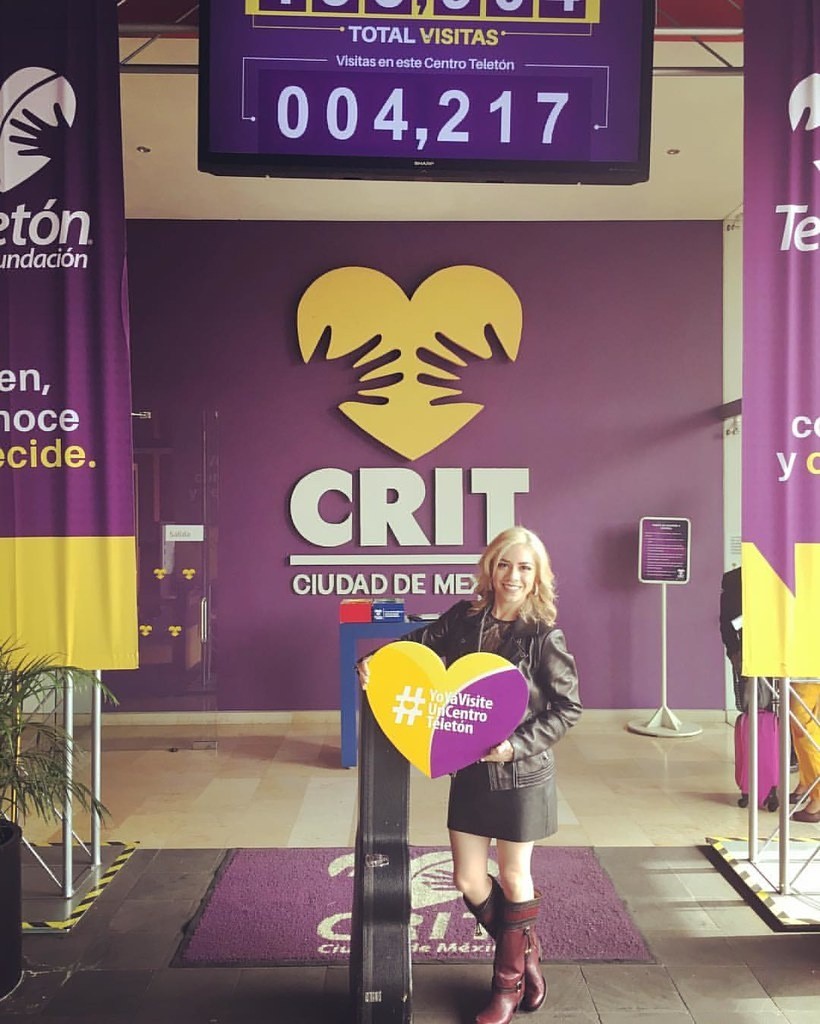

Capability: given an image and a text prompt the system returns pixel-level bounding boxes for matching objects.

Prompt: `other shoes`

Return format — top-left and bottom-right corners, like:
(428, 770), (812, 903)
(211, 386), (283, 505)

(790, 793), (808, 804)
(793, 810), (820, 822)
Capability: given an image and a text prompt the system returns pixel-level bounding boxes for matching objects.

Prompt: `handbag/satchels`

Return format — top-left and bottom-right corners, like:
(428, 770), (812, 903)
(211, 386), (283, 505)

(734, 677), (771, 712)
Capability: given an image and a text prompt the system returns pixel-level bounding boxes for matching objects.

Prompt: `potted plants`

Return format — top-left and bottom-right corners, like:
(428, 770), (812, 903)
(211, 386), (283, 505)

(1, 630), (120, 1008)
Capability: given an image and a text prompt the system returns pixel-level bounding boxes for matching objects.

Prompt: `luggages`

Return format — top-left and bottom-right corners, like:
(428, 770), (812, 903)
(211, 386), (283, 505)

(735, 709), (779, 812)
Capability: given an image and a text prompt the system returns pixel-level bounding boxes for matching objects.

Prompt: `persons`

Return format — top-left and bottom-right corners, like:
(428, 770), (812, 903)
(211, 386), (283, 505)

(355, 526), (581, 1024)
(721, 567), (820, 822)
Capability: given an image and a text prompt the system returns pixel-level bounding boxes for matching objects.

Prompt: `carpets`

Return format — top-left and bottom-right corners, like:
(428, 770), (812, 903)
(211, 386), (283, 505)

(173, 845), (652, 968)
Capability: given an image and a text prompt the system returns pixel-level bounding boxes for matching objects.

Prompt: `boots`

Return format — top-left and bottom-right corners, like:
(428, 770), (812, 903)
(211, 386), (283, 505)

(463, 874), (548, 1024)
